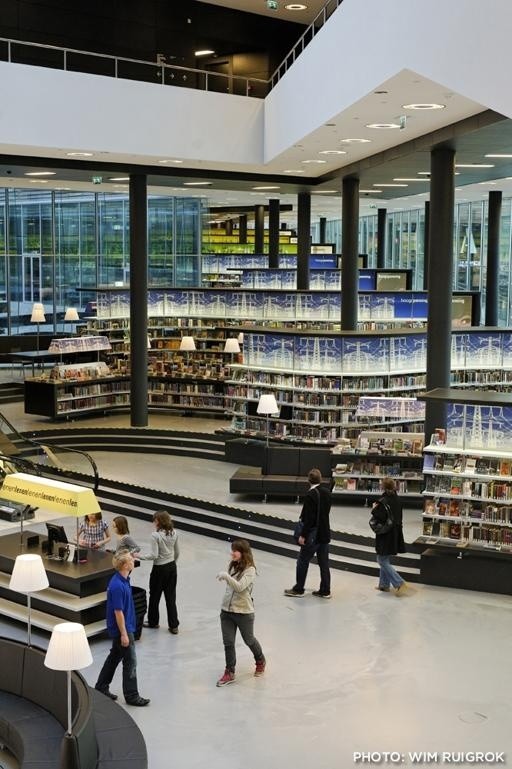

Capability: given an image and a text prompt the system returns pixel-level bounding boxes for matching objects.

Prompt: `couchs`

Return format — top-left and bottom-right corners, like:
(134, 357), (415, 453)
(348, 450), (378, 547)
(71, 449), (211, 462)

(229, 446), (332, 504)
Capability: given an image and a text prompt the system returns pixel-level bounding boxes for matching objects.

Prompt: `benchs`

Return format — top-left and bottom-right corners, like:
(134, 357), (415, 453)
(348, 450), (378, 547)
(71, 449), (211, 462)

(1, 634), (149, 769)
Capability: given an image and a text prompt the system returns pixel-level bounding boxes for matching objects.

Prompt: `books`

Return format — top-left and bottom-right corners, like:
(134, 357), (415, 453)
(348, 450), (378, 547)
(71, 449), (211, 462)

(18, 315), (511, 444)
(421, 443), (512, 553)
(330, 435), (423, 496)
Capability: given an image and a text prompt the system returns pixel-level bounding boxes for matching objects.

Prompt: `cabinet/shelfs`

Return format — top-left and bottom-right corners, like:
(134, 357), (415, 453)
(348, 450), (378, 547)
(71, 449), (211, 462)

(148, 317), (338, 445)
(339, 318), (426, 437)
(330, 439), (425, 503)
(421, 446), (512, 552)
(27, 373), (116, 418)
(87, 317), (132, 409)
(450, 331), (512, 401)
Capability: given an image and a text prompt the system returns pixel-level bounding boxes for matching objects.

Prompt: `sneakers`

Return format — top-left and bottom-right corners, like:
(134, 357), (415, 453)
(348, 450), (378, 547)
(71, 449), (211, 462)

(217, 669), (236, 687)
(395, 583), (408, 596)
(254, 661), (266, 677)
(143, 622), (159, 628)
(312, 592), (330, 598)
(127, 697), (150, 706)
(169, 628), (178, 634)
(284, 589), (304, 598)
(105, 691), (117, 700)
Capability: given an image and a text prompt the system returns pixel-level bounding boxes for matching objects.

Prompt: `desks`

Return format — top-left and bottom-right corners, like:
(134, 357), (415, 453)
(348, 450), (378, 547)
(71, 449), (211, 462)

(9, 350), (91, 379)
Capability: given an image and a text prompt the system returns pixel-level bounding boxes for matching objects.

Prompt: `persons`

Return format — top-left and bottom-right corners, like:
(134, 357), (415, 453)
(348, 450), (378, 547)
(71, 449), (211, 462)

(283, 468), (332, 599)
(215, 539), (267, 688)
(112, 515), (143, 558)
(95, 549), (151, 707)
(73, 511), (114, 552)
(371, 477), (410, 597)
(133, 510), (180, 634)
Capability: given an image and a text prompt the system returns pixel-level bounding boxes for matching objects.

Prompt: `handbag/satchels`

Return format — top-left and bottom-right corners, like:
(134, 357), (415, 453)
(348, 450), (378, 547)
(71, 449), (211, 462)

(369, 515), (394, 535)
(294, 522), (320, 545)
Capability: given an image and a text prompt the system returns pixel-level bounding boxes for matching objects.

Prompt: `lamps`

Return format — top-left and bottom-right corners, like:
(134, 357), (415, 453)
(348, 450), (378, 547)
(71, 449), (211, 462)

(223, 339), (241, 379)
(30, 301), (47, 349)
(43, 621), (95, 736)
(63, 306), (81, 338)
(9, 552), (51, 648)
(255, 393), (280, 448)
(179, 335), (195, 374)
